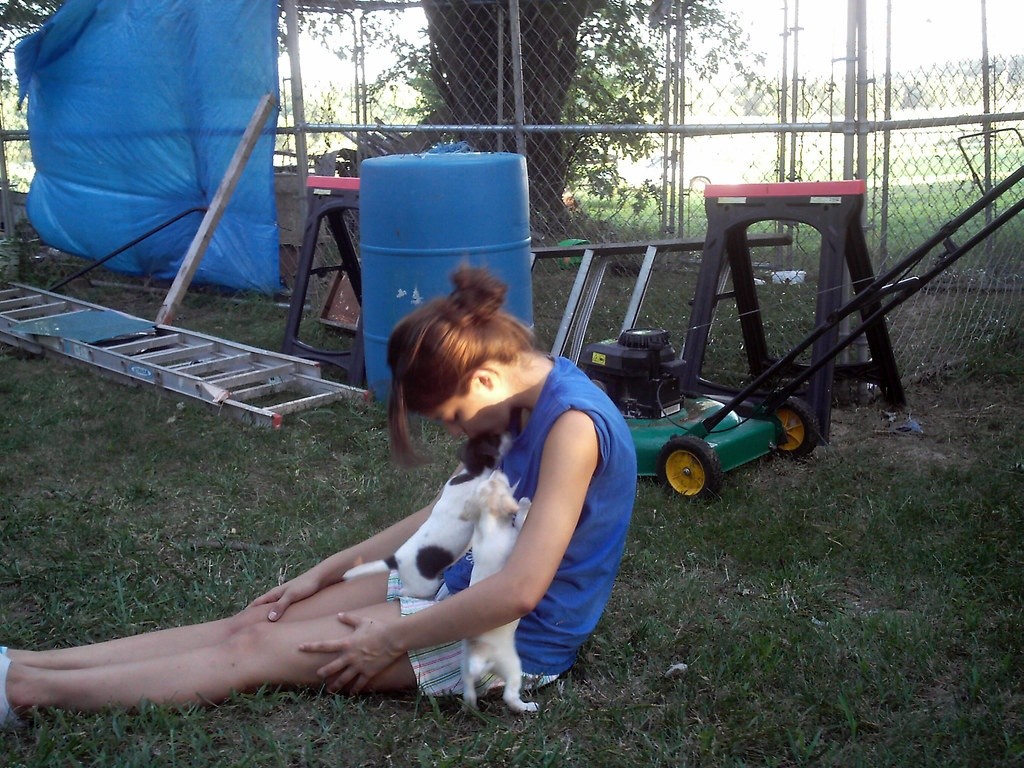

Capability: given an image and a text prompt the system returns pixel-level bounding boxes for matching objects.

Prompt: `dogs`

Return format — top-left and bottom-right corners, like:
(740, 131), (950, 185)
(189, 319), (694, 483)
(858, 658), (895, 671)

(459, 466), (541, 714)
(341, 428), (514, 601)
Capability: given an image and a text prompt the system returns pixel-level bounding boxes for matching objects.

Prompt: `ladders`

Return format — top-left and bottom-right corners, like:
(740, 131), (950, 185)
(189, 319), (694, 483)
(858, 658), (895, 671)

(0, 281), (373, 423)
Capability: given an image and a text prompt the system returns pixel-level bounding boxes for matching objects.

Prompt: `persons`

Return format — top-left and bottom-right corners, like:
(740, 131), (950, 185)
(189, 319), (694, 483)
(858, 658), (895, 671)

(1, 263), (636, 731)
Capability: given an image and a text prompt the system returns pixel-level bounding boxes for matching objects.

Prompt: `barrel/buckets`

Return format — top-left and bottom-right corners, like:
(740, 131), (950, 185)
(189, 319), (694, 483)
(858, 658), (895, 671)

(358, 152), (535, 407)
